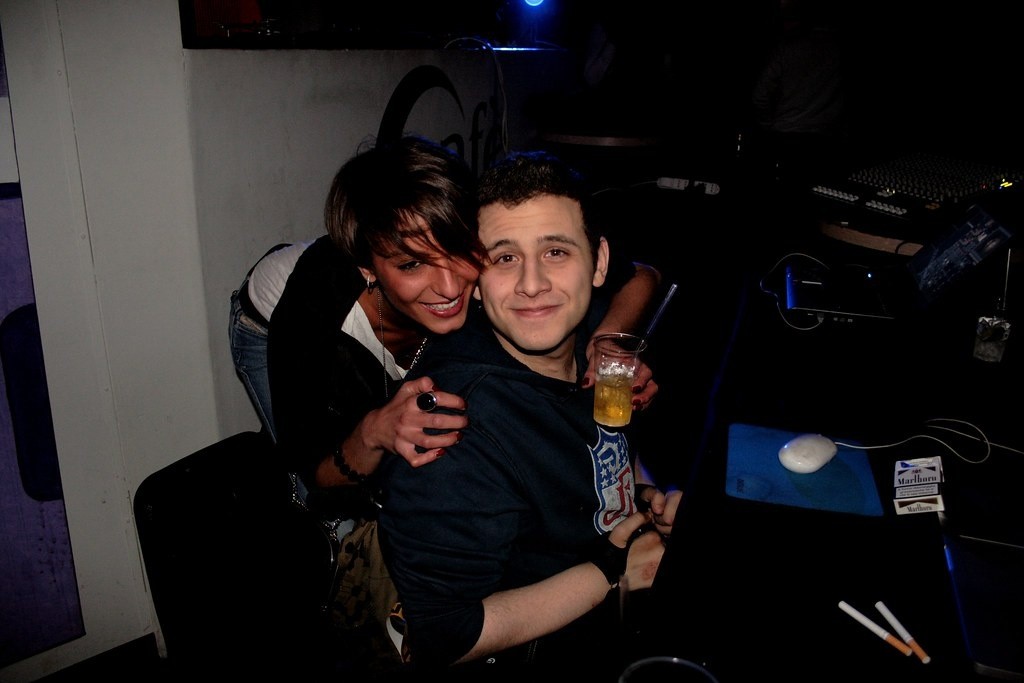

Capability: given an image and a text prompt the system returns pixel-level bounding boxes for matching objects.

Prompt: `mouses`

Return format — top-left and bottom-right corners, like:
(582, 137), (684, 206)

(778, 433), (837, 474)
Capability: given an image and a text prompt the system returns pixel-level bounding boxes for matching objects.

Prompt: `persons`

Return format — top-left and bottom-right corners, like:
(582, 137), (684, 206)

(228, 135), (654, 506)
(376, 150), (684, 669)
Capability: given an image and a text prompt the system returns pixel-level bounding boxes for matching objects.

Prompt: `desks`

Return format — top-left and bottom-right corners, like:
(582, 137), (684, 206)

(620, 267), (1024, 682)
(542, 127), (659, 153)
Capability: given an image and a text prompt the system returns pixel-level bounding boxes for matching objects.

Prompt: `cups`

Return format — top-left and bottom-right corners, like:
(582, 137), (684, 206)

(593, 333), (646, 427)
(974, 297), (1014, 362)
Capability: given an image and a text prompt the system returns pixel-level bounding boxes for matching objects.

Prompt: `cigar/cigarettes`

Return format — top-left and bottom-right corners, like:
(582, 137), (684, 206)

(838, 601), (913, 657)
(874, 600), (932, 664)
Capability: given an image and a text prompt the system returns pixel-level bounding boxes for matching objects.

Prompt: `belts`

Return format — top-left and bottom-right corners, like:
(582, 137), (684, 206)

(231, 289), (269, 337)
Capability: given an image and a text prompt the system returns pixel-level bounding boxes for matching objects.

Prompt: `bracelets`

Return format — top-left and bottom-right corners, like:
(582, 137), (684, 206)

(333, 446), (367, 484)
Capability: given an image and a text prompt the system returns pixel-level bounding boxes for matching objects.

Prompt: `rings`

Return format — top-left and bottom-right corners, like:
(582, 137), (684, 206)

(416, 394), (438, 411)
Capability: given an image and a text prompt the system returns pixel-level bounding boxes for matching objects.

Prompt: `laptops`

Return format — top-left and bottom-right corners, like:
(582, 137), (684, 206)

(786, 205), (1014, 322)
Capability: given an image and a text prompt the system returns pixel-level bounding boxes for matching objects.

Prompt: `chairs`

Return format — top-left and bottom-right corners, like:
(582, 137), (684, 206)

(130, 430), (405, 683)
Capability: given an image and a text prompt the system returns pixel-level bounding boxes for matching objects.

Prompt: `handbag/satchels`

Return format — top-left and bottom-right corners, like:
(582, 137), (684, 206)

(267, 471), (341, 612)
